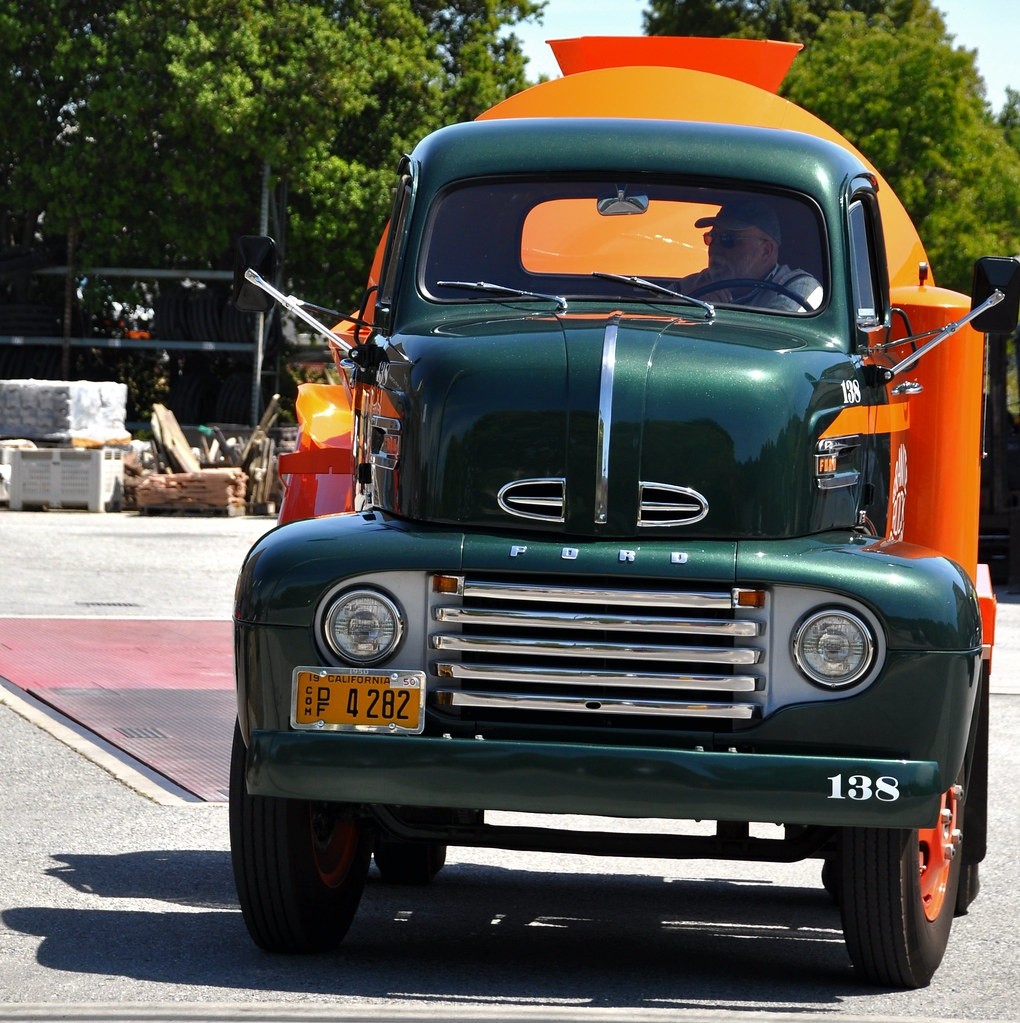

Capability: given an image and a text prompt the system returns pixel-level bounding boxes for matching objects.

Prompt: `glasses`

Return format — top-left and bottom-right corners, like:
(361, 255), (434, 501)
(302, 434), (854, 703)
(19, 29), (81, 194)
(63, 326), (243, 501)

(703, 232), (774, 249)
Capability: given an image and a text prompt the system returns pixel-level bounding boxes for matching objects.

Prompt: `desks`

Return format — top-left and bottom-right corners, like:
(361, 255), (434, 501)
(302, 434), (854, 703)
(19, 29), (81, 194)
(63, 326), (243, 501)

(9, 447), (125, 513)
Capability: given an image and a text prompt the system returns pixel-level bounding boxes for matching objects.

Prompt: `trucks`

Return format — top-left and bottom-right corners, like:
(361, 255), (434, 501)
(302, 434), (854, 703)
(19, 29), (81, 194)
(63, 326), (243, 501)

(228, 36), (1019, 991)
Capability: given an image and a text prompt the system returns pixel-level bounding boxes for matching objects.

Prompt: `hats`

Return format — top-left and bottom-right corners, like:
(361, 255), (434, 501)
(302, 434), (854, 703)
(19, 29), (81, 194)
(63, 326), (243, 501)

(694, 198), (780, 241)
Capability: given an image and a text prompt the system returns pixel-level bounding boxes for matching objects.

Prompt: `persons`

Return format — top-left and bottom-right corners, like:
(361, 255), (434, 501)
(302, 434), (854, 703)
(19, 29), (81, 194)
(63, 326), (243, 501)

(655, 195), (823, 312)
